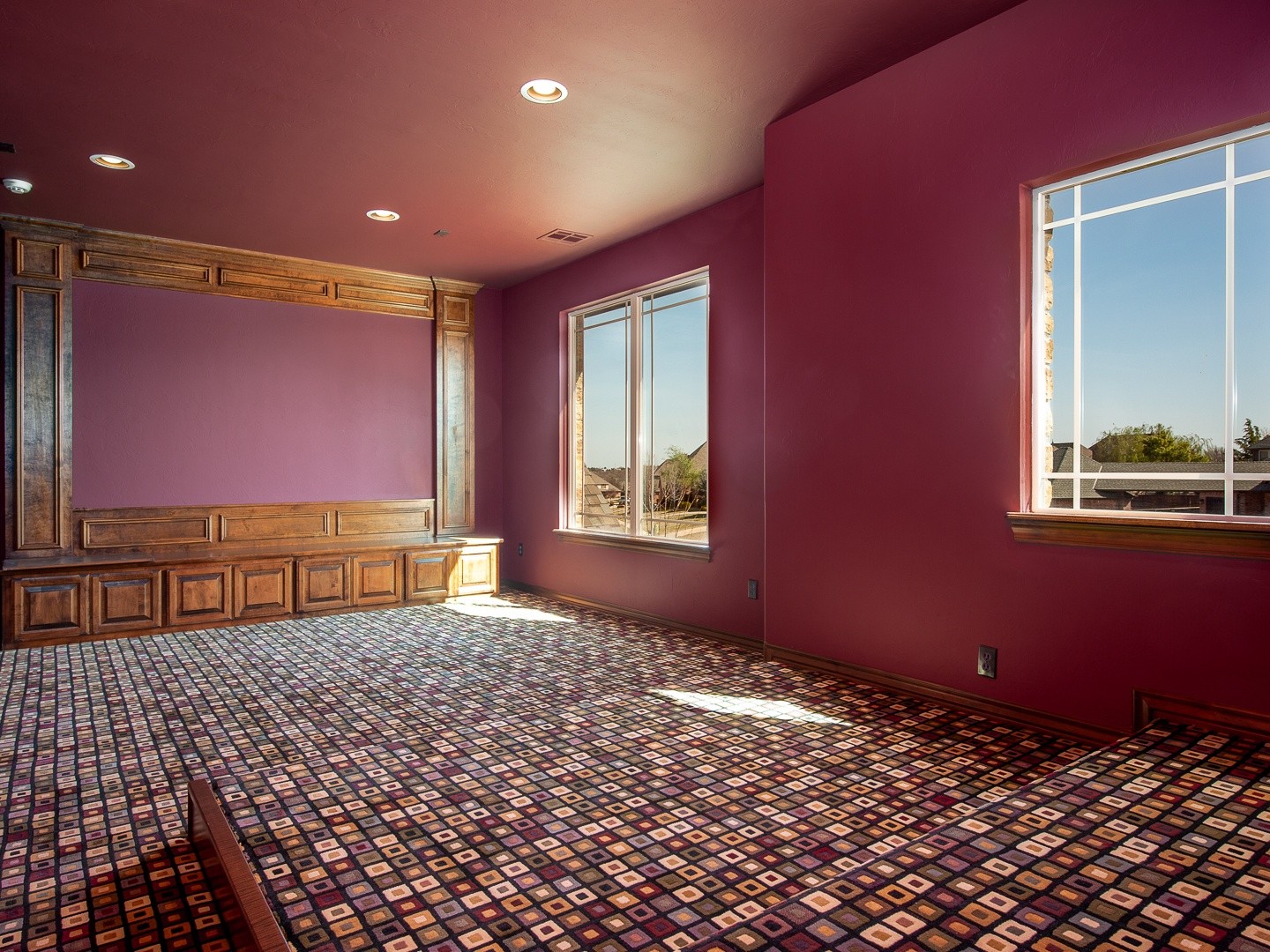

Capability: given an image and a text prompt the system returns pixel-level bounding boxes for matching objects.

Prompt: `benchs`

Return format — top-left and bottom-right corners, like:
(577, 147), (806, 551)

(0, 533), (503, 650)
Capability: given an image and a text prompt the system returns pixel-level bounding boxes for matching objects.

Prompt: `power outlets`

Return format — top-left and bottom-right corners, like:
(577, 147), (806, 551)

(977, 645), (997, 679)
(748, 580), (758, 599)
